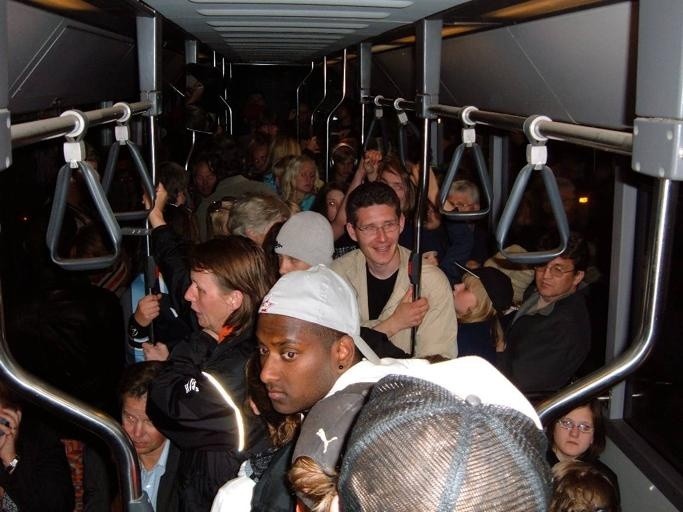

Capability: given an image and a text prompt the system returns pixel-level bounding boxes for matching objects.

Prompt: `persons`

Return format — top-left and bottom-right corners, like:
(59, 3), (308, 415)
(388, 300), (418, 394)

(0, 88), (624, 512)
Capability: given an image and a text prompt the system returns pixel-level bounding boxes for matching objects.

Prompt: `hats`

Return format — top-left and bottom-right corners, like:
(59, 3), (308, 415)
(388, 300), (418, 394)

(291, 355), (553, 512)
(274, 211), (335, 266)
(455, 244), (534, 310)
(258, 264), (382, 364)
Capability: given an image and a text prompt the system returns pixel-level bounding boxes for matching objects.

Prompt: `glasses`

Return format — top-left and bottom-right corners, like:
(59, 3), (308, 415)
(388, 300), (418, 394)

(559, 420), (594, 433)
(357, 223), (399, 234)
(537, 266), (574, 277)
